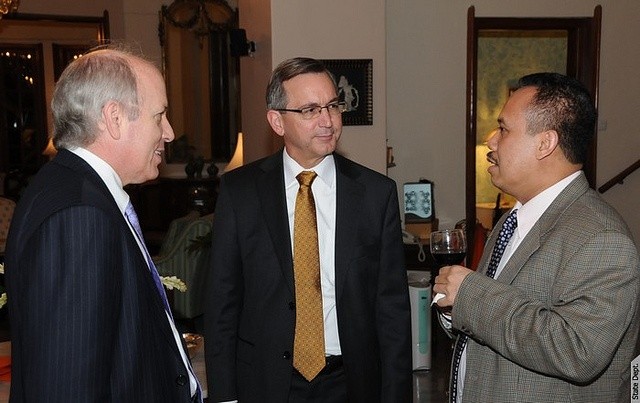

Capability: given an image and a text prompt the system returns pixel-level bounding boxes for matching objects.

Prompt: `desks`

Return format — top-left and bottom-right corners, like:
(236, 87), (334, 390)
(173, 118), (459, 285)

(399, 244), (441, 357)
(159, 174), (219, 221)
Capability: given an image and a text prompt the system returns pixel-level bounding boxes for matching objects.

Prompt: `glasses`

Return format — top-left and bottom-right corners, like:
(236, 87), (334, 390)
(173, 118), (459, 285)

(275, 99), (348, 120)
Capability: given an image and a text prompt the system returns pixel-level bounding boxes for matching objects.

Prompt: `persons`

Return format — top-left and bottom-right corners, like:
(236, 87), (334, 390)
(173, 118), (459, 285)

(201, 57), (413, 401)
(0, 36), (203, 402)
(433, 71), (640, 403)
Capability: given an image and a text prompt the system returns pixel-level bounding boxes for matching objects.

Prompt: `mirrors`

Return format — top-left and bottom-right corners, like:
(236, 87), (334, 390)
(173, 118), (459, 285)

(156, 1), (247, 166)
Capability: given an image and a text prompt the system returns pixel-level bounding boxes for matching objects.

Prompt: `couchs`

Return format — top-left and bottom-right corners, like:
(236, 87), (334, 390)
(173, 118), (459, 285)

(146, 207), (219, 321)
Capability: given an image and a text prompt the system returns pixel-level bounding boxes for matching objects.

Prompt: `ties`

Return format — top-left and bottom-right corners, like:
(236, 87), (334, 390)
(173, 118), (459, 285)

(293, 171), (326, 384)
(125, 199), (205, 402)
(450, 209), (518, 403)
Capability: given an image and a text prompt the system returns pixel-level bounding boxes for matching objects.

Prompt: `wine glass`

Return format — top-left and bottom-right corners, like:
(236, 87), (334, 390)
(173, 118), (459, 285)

(429, 229), (468, 313)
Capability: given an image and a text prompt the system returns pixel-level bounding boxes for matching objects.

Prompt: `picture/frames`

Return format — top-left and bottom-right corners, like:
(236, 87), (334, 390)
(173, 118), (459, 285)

(302, 58), (373, 126)
